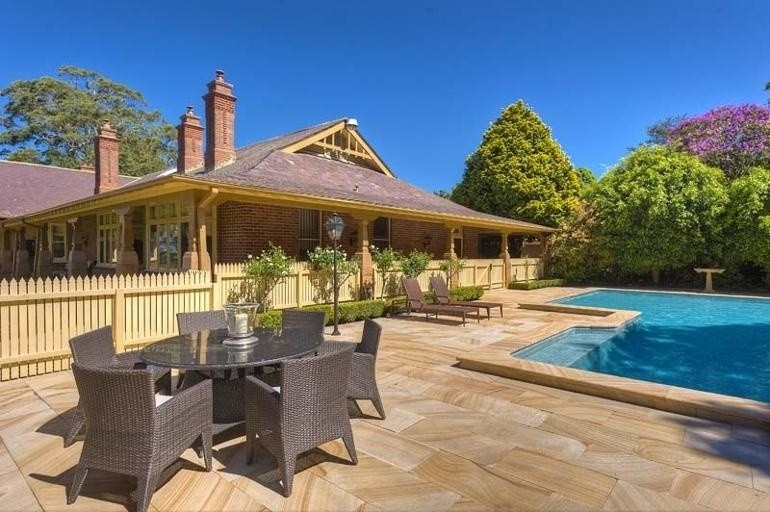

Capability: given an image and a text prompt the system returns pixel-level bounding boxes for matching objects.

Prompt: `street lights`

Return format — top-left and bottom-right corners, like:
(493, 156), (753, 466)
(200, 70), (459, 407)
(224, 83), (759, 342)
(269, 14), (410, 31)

(324, 210), (345, 336)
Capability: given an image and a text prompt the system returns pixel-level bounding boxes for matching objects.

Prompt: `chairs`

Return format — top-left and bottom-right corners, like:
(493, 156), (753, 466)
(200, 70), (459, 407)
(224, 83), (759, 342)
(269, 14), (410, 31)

(390, 278), (480, 328)
(176, 309), (229, 388)
(245, 342), (362, 498)
(67, 364), (213, 512)
(430, 276), (503, 320)
(266, 309), (325, 369)
(316, 318), (388, 421)
(66, 324), (172, 447)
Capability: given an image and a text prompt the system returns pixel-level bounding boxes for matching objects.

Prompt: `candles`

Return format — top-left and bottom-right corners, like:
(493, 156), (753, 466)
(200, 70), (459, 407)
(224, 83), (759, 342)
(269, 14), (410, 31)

(235, 314), (247, 333)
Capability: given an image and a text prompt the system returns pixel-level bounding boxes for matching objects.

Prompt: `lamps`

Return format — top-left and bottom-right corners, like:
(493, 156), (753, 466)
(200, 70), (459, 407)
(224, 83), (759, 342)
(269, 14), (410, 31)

(350, 228), (357, 246)
(422, 231), (433, 246)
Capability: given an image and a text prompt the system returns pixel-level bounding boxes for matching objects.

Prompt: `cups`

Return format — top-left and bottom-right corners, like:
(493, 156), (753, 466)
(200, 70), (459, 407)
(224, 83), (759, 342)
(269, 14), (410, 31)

(223, 302), (260, 339)
